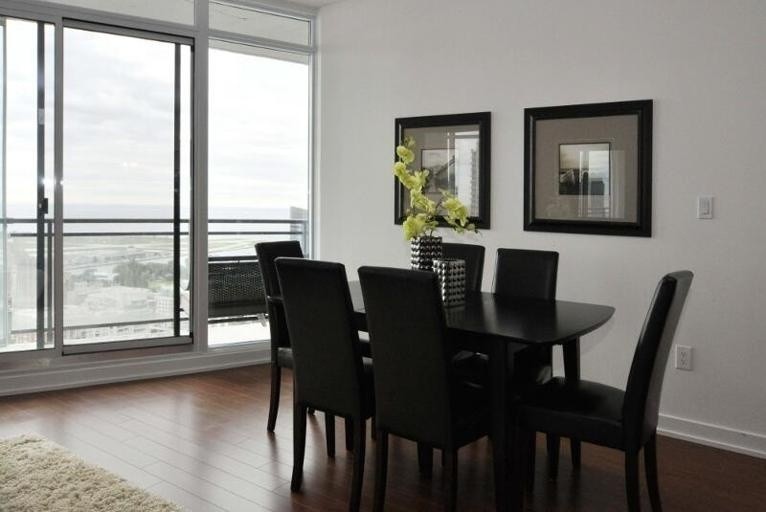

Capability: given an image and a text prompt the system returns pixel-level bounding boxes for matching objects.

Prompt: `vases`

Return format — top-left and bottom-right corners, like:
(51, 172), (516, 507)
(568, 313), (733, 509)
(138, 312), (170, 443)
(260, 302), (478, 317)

(431, 258), (469, 308)
(408, 234), (442, 273)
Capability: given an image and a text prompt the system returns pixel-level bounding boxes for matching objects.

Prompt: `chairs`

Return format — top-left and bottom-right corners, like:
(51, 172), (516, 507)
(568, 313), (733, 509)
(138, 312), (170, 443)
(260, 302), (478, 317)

(356, 265), (510, 512)
(514, 268), (694, 512)
(254, 240), (377, 451)
(443, 242), (486, 292)
(273, 256), (434, 511)
(466, 247), (560, 400)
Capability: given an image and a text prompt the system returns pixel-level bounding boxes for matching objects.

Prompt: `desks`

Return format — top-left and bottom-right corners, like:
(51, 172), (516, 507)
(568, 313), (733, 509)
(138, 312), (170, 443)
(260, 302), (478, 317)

(266, 279), (616, 512)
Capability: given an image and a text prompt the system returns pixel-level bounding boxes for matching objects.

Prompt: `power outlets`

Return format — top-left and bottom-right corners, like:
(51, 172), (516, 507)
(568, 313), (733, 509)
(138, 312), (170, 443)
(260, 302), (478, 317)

(673, 344), (694, 370)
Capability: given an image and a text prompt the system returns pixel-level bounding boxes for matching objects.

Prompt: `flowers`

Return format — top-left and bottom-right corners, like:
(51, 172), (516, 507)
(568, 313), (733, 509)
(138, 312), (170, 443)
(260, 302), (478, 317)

(392, 136), (483, 267)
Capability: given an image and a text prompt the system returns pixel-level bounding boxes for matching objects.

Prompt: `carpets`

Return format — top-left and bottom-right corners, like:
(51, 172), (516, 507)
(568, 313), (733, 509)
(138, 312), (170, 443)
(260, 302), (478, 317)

(0, 430), (184, 512)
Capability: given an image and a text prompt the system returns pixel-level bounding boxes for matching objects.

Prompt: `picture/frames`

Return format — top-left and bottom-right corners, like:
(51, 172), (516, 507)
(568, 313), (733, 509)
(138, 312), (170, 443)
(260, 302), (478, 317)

(394, 111), (492, 230)
(523, 99), (654, 238)
